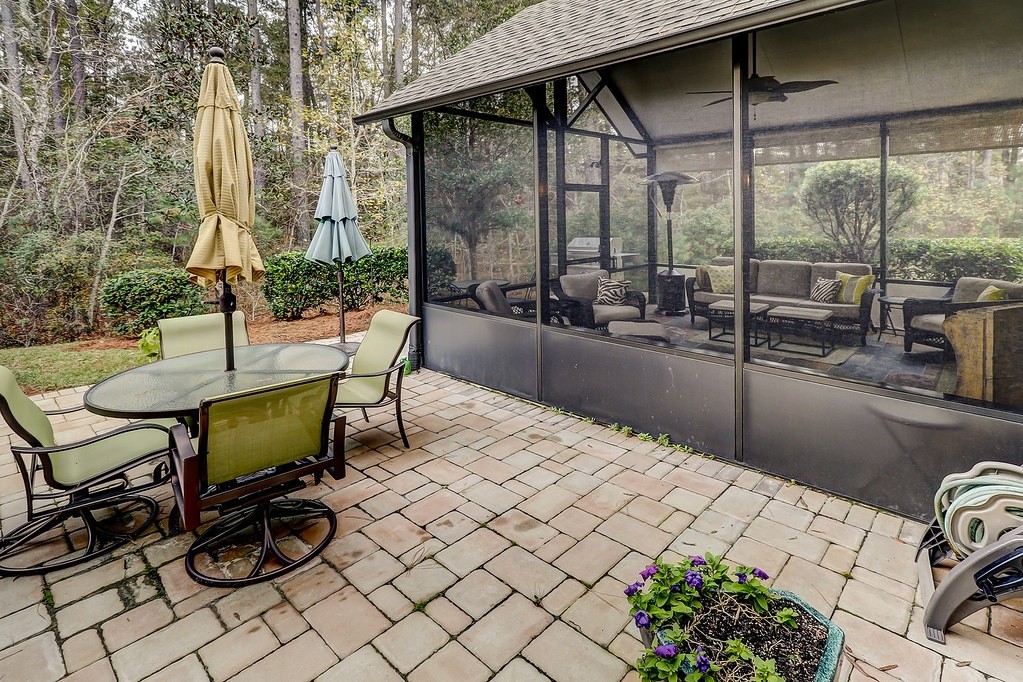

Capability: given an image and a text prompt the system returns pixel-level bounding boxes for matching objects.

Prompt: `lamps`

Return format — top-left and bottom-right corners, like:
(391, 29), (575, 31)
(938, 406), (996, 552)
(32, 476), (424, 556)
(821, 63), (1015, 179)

(748, 86), (774, 107)
(636, 169), (699, 317)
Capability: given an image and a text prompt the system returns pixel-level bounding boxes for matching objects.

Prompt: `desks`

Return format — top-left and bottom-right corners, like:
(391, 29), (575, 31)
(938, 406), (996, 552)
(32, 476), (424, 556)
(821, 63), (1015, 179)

(82, 343), (350, 537)
(450, 279), (511, 305)
(506, 296), (530, 313)
(877, 295), (909, 343)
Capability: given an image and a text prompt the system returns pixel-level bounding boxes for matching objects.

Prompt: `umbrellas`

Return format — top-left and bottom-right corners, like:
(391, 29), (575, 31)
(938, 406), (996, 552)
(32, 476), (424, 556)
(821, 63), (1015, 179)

(184, 47), (266, 369)
(304, 146), (372, 355)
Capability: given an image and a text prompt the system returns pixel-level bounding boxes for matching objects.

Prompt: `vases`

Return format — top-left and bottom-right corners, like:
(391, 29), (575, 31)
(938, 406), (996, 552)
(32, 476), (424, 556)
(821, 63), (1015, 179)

(639, 586), (846, 682)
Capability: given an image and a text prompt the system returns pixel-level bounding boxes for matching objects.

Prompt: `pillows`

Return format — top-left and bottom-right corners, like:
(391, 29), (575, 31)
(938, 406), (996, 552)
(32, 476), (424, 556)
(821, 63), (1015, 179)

(592, 275), (633, 306)
(976, 285), (1007, 301)
(704, 263), (734, 294)
(835, 269), (875, 305)
(695, 264), (711, 291)
(809, 274), (843, 303)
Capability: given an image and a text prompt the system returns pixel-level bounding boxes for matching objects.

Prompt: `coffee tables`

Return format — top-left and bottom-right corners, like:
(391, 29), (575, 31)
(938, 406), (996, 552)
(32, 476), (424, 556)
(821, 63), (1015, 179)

(708, 299), (769, 348)
(765, 305), (837, 357)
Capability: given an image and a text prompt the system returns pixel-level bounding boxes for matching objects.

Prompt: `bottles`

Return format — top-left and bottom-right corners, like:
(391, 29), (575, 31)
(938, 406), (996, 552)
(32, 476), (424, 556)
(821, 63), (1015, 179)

(399, 356), (411, 375)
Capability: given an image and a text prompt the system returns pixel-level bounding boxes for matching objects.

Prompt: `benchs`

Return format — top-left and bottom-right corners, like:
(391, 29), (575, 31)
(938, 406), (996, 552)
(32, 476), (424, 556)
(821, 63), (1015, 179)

(684, 256), (884, 347)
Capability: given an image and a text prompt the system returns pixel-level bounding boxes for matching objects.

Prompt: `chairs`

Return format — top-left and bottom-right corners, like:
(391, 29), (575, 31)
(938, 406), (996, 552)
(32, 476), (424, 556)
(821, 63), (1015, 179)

(0, 364), (181, 579)
(525, 271), (536, 300)
(560, 298), (671, 344)
(321, 308), (423, 479)
(428, 282), (463, 305)
(550, 269), (647, 333)
(168, 370), (349, 589)
(466, 282), (564, 325)
(903, 276), (1023, 364)
(156, 309), (251, 439)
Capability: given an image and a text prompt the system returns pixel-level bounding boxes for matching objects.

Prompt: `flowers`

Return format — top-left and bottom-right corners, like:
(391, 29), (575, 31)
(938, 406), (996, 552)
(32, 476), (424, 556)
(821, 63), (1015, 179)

(623, 552), (800, 682)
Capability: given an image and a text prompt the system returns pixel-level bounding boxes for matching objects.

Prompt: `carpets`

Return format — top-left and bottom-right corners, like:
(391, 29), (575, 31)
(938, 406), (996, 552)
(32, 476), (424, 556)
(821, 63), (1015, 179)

(687, 326), (860, 366)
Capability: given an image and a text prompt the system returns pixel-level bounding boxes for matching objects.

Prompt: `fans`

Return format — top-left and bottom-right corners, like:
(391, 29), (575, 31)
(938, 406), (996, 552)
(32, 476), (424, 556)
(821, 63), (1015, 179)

(684, 32), (840, 109)
